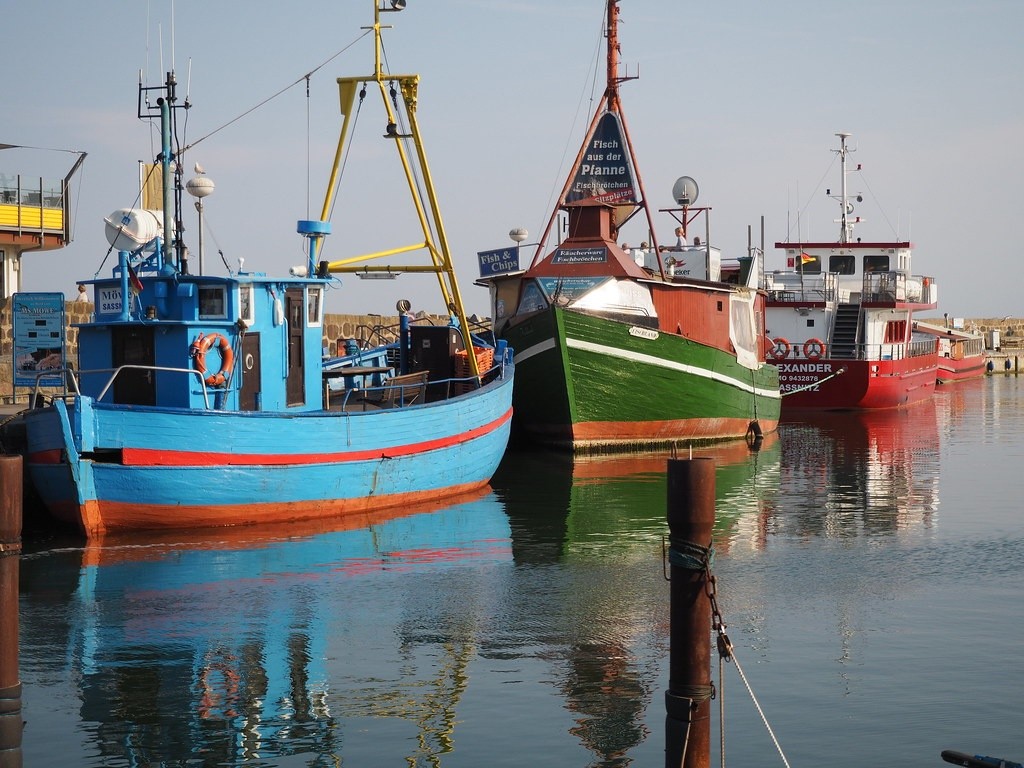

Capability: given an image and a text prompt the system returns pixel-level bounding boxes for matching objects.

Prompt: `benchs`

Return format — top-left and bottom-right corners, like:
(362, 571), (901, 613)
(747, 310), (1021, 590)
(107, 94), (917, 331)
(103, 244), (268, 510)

(1000, 336), (1024, 349)
(778, 291), (796, 302)
(0, 394), (28, 405)
(356, 370), (430, 412)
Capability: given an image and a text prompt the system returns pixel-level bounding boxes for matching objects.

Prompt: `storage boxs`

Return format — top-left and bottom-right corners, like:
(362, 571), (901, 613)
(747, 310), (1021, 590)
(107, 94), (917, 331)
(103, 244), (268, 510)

(453, 346), (494, 396)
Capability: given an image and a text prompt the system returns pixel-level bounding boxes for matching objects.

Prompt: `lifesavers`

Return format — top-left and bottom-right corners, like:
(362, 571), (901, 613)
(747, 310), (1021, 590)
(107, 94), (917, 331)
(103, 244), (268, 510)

(803, 338), (825, 361)
(769, 338), (790, 361)
(195, 333), (234, 387)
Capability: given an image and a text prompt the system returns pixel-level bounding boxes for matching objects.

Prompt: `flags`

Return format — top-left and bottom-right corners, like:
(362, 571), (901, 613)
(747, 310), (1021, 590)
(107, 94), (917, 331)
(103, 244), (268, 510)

(801, 251), (816, 264)
(126, 260), (143, 296)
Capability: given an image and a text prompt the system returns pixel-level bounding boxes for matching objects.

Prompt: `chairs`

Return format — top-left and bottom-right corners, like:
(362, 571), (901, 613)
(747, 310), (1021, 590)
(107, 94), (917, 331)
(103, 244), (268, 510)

(43, 197), (62, 208)
(0, 191), (16, 204)
(24, 193), (40, 206)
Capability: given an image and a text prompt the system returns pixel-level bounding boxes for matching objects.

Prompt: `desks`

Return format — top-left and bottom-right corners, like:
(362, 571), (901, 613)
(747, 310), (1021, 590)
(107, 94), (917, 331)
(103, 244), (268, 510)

(322, 366), (393, 411)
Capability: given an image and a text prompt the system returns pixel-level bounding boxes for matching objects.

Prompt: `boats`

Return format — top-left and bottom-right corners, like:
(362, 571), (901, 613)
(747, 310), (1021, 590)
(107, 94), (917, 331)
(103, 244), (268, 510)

(476, 431), (787, 574)
(0, 479), (517, 768)
(20, 1), (518, 542)
(910, 320), (989, 385)
(474, 2), (782, 455)
(759, 129), (942, 412)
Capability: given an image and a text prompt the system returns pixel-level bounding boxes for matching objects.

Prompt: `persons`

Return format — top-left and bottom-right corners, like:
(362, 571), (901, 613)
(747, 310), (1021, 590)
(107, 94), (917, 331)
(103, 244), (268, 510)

(146, 307), (159, 321)
(622, 243), (631, 254)
(640, 242), (648, 253)
(76, 285), (88, 302)
(923, 277), (928, 286)
(689, 237), (706, 252)
(666, 256), (677, 275)
(675, 227), (687, 250)
